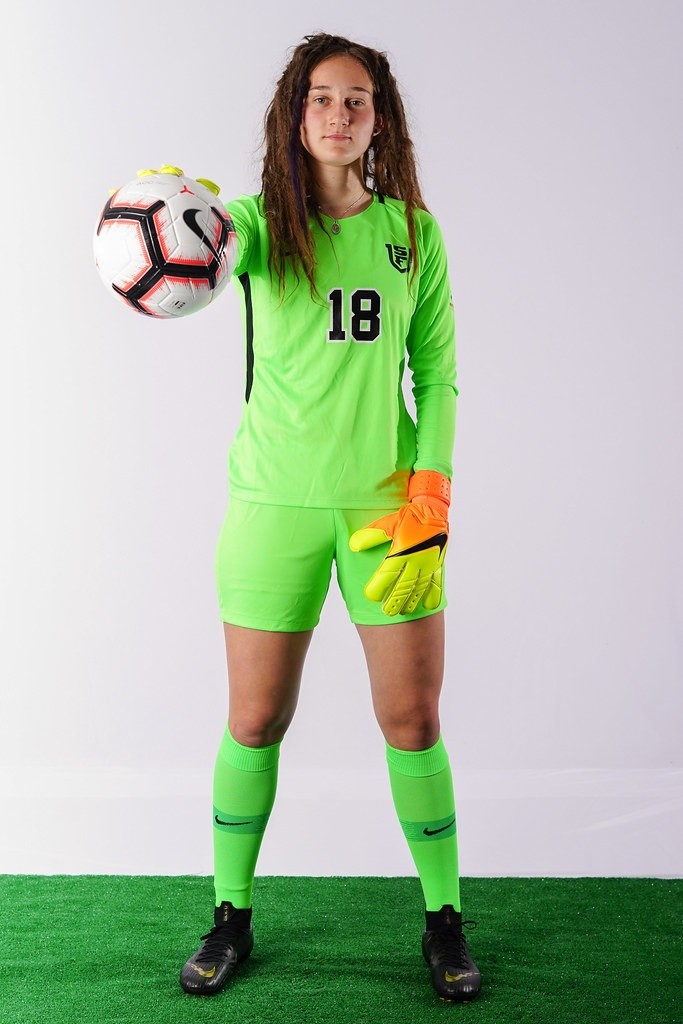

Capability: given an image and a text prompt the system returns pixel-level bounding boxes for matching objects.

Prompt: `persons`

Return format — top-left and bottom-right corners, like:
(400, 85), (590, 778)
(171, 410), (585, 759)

(109, 32), (482, 1003)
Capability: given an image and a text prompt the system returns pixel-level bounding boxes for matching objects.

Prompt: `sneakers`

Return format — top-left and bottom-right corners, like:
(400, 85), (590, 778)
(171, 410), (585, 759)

(178, 924), (255, 994)
(421, 928), (482, 1004)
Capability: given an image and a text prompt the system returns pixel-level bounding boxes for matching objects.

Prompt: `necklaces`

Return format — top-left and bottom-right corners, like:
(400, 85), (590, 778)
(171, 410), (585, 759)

(317, 185), (366, 234)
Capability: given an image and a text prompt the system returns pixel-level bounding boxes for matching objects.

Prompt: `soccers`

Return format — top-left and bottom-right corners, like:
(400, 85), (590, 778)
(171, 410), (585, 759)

(95, 174), (238, 319)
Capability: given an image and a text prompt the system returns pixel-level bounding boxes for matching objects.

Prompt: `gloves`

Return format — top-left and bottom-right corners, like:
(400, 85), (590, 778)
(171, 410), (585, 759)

(108, 165), (220, 199)
(348, 469), (450, 619)
